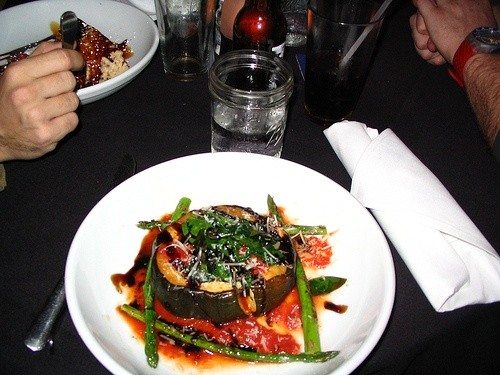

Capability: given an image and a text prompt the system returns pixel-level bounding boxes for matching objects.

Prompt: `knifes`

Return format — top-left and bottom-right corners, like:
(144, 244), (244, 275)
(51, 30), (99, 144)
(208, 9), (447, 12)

(22, 151), (137, 354)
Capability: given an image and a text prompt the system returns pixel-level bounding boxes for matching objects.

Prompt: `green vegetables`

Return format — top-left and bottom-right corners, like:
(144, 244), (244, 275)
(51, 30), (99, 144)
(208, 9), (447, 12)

(180, 212), (347, 297)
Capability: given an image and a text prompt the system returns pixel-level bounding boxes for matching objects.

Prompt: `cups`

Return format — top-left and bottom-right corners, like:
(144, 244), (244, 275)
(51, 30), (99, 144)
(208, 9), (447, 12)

(281, 0), (307, 48)
(209, 49), (294, 159)
(154, 0), (216, 81)
(304, 0), (384, 127)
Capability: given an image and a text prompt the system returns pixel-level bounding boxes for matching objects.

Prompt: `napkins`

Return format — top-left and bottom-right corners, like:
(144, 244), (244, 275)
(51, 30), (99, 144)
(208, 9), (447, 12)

(323, 120), (500, 312)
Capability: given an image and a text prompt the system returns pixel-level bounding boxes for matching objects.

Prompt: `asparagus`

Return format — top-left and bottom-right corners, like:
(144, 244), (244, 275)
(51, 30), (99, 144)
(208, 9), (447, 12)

(121, 304), (341, 363)
(138, 220), (326, 236)
(144, 197), (192, 368)
(266, 195), (321, 354)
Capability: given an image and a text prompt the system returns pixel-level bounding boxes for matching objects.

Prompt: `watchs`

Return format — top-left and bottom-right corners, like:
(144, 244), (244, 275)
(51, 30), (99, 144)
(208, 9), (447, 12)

(448, 26), (500, 87)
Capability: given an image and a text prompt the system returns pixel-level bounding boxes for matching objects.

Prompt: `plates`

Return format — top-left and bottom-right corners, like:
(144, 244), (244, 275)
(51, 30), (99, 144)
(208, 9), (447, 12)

(64, 152), (396, 375)
(130, 0), (168, 20)
(0, 0), (160, 106)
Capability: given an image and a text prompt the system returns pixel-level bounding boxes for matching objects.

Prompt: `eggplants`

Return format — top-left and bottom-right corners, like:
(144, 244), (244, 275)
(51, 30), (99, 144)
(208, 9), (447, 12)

(149, 205), (294, 321)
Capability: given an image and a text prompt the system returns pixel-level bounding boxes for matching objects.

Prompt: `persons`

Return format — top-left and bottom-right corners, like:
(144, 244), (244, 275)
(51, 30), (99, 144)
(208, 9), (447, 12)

(410, 0), (500, 162)
(0, 41), (87, 191)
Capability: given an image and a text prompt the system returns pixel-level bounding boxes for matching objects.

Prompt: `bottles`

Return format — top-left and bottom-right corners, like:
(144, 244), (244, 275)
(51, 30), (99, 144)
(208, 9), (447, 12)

(233, 0), (287, 92)
